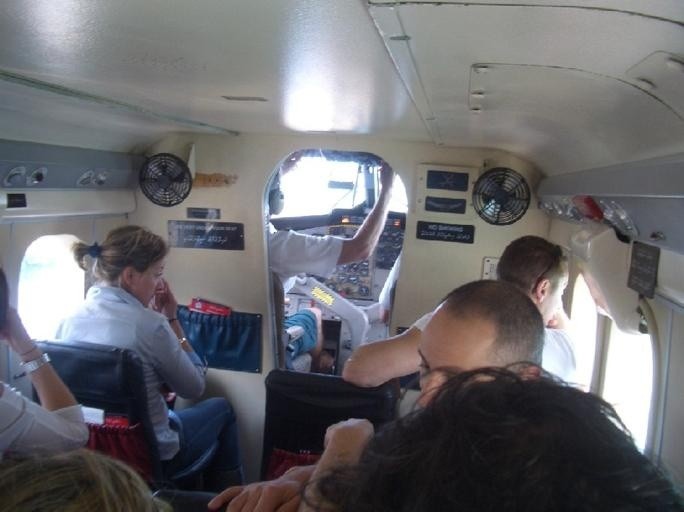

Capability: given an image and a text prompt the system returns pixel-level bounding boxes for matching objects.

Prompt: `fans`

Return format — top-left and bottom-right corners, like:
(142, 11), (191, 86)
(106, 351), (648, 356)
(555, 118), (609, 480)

(470, 166), (532, 226)
(138, 153), (193, 207)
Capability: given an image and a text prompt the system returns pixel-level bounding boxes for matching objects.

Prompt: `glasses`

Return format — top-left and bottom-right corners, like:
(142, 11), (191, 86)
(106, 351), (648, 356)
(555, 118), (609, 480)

(533, 242), (563, 292)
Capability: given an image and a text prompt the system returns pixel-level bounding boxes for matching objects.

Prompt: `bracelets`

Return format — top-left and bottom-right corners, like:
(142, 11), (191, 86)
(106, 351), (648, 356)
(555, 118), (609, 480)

(178, 337), (186, 345)
(166, 318), (177, 323)
(11, 341), (40, 361)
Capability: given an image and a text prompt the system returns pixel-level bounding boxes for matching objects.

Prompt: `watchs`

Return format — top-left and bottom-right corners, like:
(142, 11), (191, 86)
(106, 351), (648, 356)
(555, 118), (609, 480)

(24, 354), (52, 377)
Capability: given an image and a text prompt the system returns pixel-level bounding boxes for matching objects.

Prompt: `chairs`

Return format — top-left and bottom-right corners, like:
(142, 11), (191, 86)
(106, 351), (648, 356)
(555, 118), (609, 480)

(259, 369), (397, 482)
(31, 339), (224, 493)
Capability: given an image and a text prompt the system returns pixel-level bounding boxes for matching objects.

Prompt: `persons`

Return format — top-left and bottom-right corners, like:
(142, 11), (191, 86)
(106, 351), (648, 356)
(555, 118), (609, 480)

(0, 272), (89, 454)
(0, 452), (171, 512)
(343, 235), (576, 387)
(208, 279), (544, 512)
(263, 151), (393, 372)
(53, 224), (236, 490)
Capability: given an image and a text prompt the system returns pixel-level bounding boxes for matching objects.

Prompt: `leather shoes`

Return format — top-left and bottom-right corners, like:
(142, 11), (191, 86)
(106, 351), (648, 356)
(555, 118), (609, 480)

(315, 352), (334, 373)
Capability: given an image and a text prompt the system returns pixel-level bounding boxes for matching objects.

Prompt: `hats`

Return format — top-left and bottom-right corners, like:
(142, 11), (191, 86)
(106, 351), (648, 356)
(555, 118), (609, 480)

(270, 170), (280, 190)
